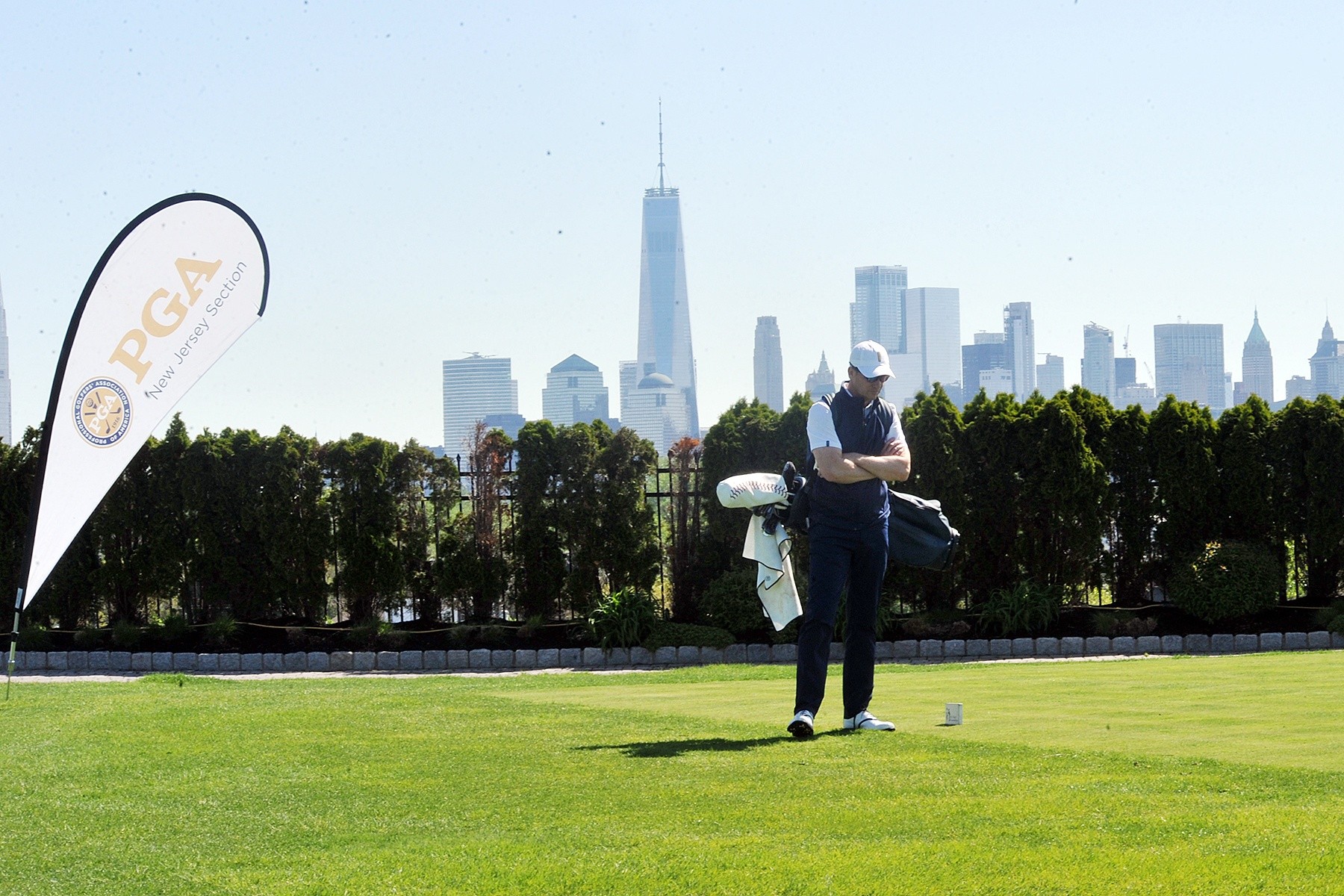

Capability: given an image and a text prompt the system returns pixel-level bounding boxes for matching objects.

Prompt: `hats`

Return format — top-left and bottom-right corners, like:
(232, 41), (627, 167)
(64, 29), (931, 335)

(849, 340), (896, 379)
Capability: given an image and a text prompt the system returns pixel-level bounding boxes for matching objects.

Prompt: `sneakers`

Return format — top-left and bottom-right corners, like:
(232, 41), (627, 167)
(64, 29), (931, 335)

(787, 710), (814, 738)
(842, 708), (896, 731)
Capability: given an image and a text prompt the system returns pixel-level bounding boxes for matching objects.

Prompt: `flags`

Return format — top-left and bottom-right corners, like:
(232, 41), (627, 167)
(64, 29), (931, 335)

(14, 192), (269, 614)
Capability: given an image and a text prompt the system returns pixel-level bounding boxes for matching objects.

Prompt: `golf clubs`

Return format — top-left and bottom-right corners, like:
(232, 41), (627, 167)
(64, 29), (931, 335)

(751, 461), (807, 537)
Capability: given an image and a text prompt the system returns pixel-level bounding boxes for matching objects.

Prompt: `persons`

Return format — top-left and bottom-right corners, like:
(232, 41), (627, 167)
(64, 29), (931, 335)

(786, 338), (912, 743)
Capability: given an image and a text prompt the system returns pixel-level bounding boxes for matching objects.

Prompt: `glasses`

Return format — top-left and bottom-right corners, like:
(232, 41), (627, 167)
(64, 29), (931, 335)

(867, 375), (890, 383)
